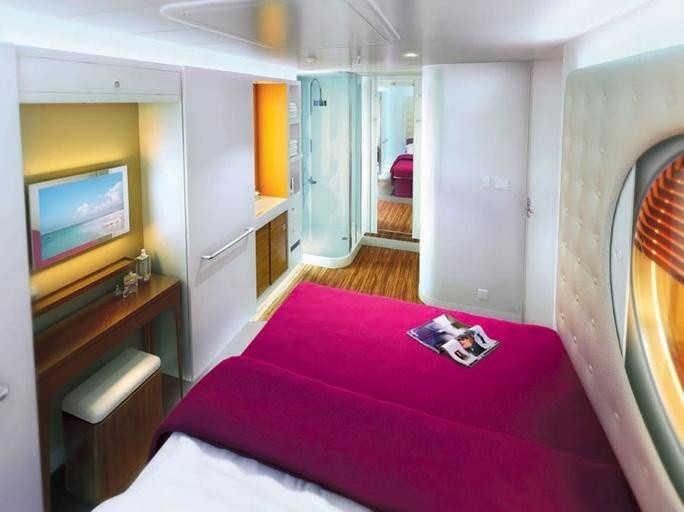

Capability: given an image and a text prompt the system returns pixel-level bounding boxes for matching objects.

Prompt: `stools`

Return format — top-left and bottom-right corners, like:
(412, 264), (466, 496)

(62, 347), (161, 507)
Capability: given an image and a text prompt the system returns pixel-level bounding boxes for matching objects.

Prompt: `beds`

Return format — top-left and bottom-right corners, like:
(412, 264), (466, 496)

(389, 138), (414, 198)
(85, 280), (645, 511)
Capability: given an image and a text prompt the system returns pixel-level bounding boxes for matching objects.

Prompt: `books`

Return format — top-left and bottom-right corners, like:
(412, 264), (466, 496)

(406, 314), (503, 367)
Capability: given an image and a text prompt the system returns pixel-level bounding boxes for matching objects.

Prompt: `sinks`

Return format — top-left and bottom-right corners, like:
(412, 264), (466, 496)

(255, 198), (279, 217)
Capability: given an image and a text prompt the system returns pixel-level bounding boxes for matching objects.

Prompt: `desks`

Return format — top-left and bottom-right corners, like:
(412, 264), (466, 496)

(34, 265), (182, 512)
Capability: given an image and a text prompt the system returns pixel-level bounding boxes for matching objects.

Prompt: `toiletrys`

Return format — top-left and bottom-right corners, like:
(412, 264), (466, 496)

(135, 248), (151, 283)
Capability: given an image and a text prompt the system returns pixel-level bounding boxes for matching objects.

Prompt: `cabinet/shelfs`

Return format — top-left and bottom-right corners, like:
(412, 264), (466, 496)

(256, 80), (303, 199)
(256, 210), (288, 299)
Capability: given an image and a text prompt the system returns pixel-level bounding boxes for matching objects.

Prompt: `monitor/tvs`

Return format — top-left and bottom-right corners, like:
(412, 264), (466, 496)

(27, 164), (130, 273)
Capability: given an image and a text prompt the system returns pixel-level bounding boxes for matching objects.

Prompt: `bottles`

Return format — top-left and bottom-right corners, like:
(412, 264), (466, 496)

(123, 249), (152, 297)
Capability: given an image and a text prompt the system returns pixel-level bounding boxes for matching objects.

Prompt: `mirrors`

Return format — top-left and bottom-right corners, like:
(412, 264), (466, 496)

(377, 76), (414, 235)
